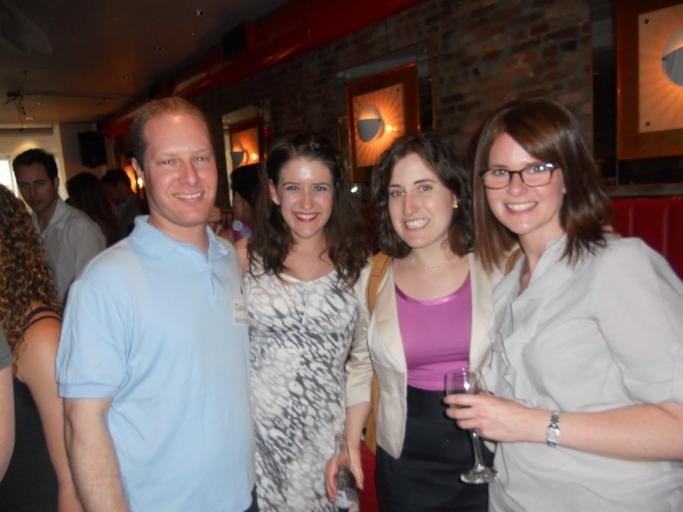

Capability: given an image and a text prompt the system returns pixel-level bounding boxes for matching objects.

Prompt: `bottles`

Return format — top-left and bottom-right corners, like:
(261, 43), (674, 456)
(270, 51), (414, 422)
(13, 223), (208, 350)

(330, 431), (359, 512)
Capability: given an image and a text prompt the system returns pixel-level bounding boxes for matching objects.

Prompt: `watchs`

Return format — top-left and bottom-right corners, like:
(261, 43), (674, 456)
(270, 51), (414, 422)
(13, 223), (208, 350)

(547, 410), (560, 448)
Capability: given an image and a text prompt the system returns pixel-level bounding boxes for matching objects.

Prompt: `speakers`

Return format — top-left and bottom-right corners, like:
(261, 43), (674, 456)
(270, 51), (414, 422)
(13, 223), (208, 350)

(78, 130), (108, 168)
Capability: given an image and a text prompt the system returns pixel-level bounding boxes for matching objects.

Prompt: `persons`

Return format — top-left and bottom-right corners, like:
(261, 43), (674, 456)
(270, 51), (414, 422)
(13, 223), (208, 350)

(439, 99), (682, 512)
(0, 184), (87, 512)
(235, 132), (376, 512)
(324, 129), (509, 511)
(13, 147), (106, 308)
(55, 93), (257, 511)
(64, 146), (260, 309)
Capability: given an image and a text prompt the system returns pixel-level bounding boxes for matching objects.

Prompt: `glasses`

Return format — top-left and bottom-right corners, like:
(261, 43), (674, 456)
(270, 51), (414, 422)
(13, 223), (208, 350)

(479, 161), (561, 189)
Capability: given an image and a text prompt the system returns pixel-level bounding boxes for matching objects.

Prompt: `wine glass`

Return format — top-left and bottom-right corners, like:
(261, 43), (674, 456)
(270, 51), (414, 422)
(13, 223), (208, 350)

(442, 369), (500, 488)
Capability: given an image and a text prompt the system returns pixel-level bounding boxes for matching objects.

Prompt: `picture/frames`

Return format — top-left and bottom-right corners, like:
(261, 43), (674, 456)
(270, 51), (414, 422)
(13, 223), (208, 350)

(217, 97), (272, 208)
(590, 1), (683, 197)
(335, 40), (433, 203)
